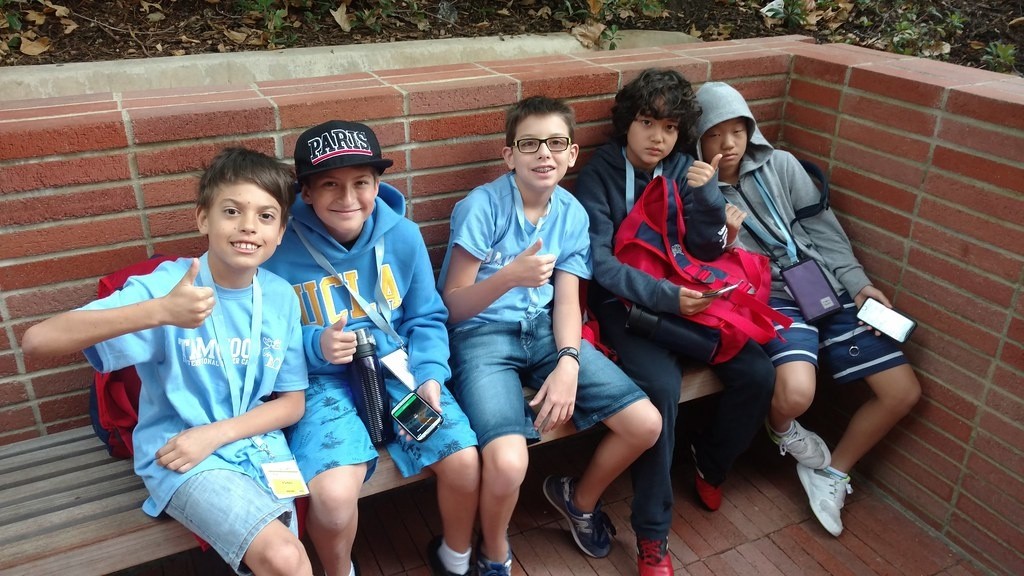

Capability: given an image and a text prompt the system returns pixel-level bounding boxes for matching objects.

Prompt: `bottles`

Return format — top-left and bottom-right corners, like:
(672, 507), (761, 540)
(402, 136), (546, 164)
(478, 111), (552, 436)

(625, 304), (721, 364)
(348, 328), (397, 445)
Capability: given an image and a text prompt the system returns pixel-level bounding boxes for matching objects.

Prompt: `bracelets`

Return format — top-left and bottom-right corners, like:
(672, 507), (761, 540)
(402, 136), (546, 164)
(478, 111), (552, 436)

(556, 347), (580, 365)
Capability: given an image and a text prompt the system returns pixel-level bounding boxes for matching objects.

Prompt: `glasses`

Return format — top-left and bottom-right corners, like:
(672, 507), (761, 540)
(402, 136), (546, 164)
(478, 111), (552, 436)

(507, 136), (570, 154)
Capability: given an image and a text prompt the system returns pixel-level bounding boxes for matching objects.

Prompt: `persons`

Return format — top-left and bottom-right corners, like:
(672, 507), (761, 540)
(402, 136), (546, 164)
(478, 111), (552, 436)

(573, 68), (776, 576)
(437, 97), (664, 576)
(258, 119), (480, 576)
(694, 81), (920, 537)
(21, 145), (316, 576)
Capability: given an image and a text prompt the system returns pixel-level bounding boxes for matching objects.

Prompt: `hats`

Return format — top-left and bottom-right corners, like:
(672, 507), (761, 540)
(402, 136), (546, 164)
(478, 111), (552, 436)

(294, 120), (393, 187)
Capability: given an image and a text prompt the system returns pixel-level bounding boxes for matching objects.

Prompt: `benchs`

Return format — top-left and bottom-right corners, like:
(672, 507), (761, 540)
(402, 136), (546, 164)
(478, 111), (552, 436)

(0, 356), (727, 576)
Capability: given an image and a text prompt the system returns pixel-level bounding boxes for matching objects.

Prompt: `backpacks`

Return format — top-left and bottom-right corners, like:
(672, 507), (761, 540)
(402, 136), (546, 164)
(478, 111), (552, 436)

(613, 175), (778, 365)
(87, 253), (196, 460)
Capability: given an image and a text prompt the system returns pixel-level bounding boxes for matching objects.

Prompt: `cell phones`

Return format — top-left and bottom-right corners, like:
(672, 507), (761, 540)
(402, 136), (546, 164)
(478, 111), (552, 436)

(702, 285), (738, 297)
(856, 296), (918, 344)
(391, 392), (443, 443)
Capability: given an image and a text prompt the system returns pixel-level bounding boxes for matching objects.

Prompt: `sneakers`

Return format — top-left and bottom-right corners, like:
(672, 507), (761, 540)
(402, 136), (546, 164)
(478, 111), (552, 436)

(427, 534), (472, 576)
(476, 528), (512, 576)
(796, 462), (853, 537)
(638, 533), (675, 576)
(543, 474), (616, 559)
(765, 414), (831, 470)
(690, 444), (721, 514)
(324, 558), (359, 576)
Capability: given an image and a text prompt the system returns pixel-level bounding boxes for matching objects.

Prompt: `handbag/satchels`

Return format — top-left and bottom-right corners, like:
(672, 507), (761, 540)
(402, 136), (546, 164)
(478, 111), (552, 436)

(780, 257), (843, 324)
